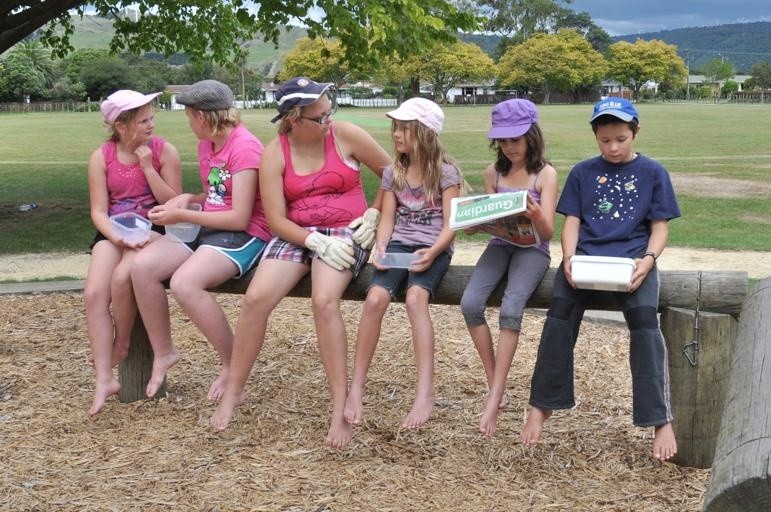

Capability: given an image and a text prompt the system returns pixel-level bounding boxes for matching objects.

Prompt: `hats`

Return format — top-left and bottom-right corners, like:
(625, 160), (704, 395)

(276, 75), (334, 114)
(487, 98), (539, 139)
(386, 97), (445, 136)
(589, 96), (639, 125)
(174, 79), (236, 110)
(99, 89), (163, 125)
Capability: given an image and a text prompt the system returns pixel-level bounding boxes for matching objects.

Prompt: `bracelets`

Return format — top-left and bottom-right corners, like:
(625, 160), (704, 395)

(639, 251), (659, 263)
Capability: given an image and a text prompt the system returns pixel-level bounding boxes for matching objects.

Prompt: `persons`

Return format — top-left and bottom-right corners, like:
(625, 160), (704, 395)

(210, 77), (394, 446)
(84, 91), (185, 417)
(458, 96), (558, 433)
(345, 95), (462, 428)
(520, 96), (681, 464)
(130, 80), (270, 409)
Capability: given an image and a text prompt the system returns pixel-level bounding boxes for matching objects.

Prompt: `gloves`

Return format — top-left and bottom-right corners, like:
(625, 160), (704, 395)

(305, 231), (356, 272)
(347, 207), (382, 250)
